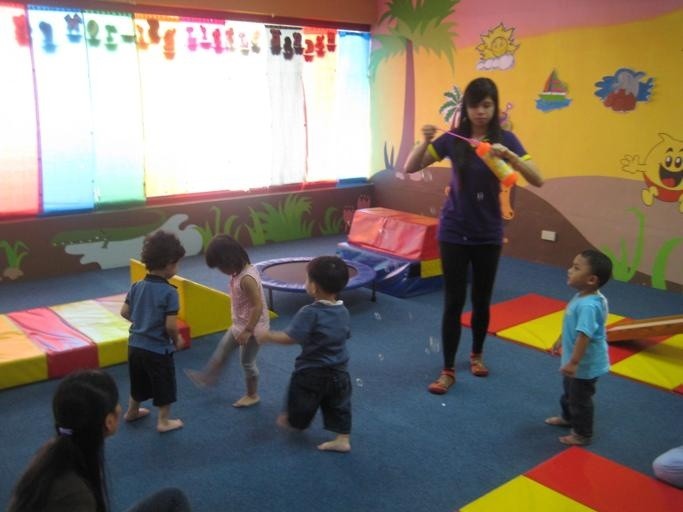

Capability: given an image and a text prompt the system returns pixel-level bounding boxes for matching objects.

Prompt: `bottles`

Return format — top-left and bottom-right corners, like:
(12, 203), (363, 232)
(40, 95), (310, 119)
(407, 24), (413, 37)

(474, 141), (518, 187)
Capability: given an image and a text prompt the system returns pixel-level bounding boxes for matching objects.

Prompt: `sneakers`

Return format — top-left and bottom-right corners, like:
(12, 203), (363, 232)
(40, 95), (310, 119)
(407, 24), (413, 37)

(427, 367), (457, 396)
(466, 351), (489, 377)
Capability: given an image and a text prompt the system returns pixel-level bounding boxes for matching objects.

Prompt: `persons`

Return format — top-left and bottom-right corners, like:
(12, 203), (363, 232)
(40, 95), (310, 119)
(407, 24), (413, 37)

(186, 234), (270, 408)
(4, 368), (189, 512)
(403, 78), (542, 395)
(254, 256), (353, 453)
(120, 230), (184, 431)
(544, 250), (615, 446)
